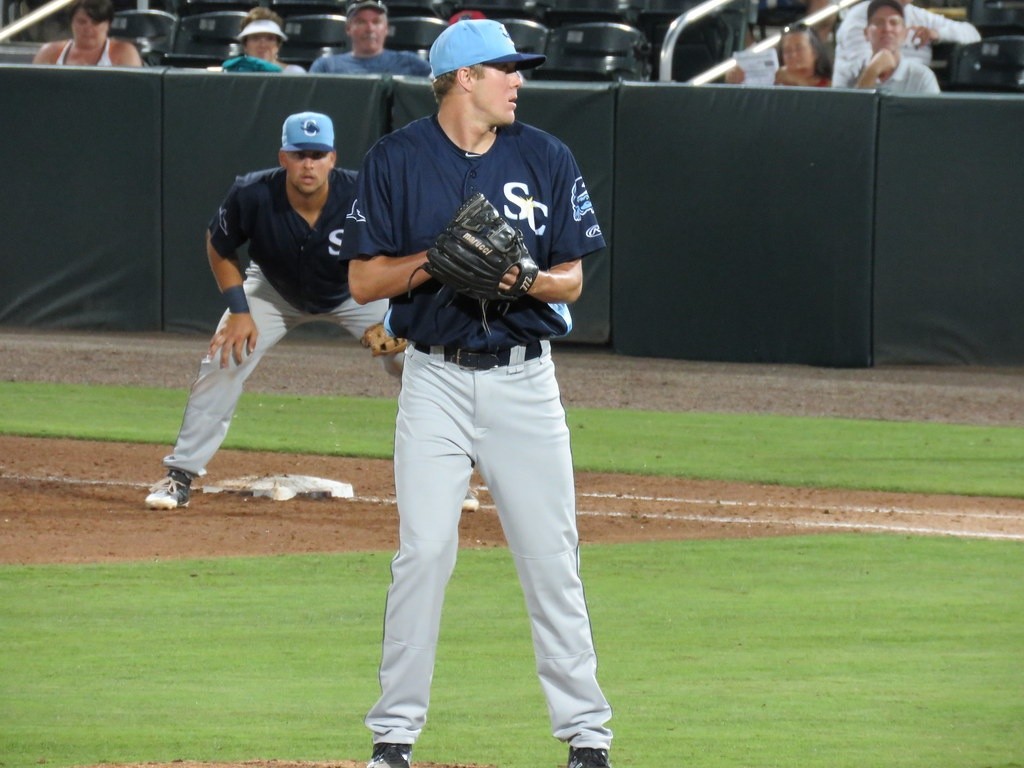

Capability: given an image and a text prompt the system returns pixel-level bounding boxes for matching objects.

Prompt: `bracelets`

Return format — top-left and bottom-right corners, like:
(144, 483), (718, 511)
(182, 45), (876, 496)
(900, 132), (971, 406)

(223, 285), (251, 314)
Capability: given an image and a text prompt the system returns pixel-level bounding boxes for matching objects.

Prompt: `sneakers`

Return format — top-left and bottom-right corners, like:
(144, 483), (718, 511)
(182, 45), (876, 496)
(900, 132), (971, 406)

(567, 746), (611, 768)
(462, 486), (479, 511)
(368, 742), (412, 768)
(145, 475), (189, 511)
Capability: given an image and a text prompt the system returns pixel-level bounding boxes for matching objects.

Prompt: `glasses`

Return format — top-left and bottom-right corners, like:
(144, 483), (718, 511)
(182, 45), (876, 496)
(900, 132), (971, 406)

(782, 23), (808, 33)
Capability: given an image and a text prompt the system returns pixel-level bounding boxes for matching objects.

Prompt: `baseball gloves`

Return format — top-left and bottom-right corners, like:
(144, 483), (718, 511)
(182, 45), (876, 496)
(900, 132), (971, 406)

(404, 190), (542, 336)
(360, 322), (410, 358)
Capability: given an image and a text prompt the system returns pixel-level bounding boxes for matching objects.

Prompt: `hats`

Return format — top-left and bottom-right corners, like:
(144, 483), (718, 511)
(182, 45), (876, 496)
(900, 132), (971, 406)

(429, 18), (547, 78)
(867, 0), (904, 24)
(341, 0), (388, 25)
(280, 111), (335, 152)
(237, 19), (288, 42)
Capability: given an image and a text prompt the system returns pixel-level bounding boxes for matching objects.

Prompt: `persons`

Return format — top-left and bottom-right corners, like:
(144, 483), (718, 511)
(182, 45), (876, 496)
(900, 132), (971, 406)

(220, 6), (306, 74)
(143, 111), (480, 511)
(309, 0), (432, 77)
(338, 18), (613, 768)
(724, 0), (981, 95)
(32, 0), (142, 67)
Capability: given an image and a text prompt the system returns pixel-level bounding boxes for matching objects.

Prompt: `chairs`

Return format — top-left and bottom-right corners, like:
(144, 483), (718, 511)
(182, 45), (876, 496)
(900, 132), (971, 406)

(108, 0), (1024, 93)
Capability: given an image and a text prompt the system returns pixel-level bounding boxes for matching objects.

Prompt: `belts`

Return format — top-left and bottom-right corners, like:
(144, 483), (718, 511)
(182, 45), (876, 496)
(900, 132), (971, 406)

(415, 341), (542, 371)
